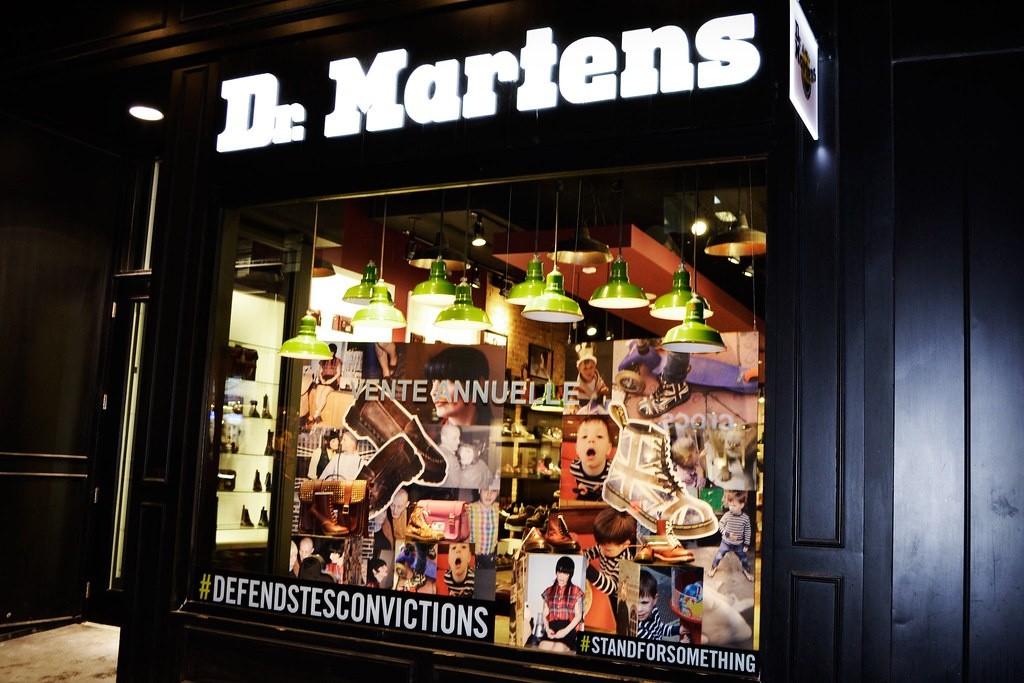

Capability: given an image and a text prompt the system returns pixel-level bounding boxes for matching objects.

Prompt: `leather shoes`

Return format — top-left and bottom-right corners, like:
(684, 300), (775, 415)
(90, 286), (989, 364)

(634, 544), (654, 561)
(344, 383), (447, 486)
(522, 526), (550, 552)
(355, 435), (424, 519)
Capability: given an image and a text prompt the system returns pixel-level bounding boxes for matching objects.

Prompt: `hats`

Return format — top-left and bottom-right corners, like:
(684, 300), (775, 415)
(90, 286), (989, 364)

(576, 347), (598, 368)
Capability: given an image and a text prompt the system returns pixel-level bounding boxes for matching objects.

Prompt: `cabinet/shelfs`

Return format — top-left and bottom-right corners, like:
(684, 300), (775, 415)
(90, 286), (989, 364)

(189, 207), (306, 572)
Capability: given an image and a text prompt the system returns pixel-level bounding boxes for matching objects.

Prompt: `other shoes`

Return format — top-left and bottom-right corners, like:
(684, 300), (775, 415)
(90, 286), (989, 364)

(389, 354), (398, 376)
(264, 430), (275, 455)
(307, 415), (323, 425)
(410, 572), (426, 588)
(403, 506), (447, 541)
(496, 417), (561, 571)
(428, 547), (436, 558)
(708, 566), (717, 578)
(742, 569), (754, 583)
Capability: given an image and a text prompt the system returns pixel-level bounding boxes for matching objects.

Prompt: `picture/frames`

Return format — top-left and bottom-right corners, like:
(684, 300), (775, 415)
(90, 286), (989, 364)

(527, 342), (554, 381)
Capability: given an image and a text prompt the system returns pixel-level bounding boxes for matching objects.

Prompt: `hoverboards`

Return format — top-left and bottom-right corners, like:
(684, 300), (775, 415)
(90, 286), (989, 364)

(614, 339), (758, 396)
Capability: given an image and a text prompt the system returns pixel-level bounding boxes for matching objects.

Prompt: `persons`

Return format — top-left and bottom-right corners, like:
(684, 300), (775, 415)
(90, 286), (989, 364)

(307, 426), (364, 480)
(569, 342), (690, 420)
(671, 436), (754, 582)
(569, 415), (621, 501)
(374, 342), (398, 391)
(424, 345), (494, 426)
(438, 423), (493, 488)
(313, 343), (343, 426)
(288, 537), (344, 585)
(584, 507), (637, 618)
(382, 488), (499, 600)
(538, 556), (585, 655)
(637, 569), (691, 644)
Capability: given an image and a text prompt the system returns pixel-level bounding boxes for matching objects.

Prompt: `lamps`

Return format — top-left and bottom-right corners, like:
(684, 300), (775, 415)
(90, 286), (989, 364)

(126, 68), (171, 122)
(506, 184), (548, 305)
(520, 192), (584, 323)
(588, 172), (648, 309)
(434, 206), (491, 331)
(649, 192), (714, 320)
(660, 192), (727, 354)
(412, 196), (456, 305)
(350, 195), (407, 329)
(277, 203), (333, 360)
(342, 216), (395, 307)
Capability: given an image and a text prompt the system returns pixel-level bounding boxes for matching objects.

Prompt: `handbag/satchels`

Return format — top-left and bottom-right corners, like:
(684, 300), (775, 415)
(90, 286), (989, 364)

(298, 474), (368, 535)
(418, 499), (470, 539)
(228, 345), (259, 381)
(217, 469), (236, 491)
(209, 418), (241, 454)
(210, 393), (244, 414)
(524, 618), (547, 648)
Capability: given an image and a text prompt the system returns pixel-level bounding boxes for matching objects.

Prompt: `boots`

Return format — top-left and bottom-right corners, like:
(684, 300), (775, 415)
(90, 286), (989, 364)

(603, 423), (720, 537)
(241, 505), (270, 527)
(249, 394), (273, 418)
(253, 470), (274, 492)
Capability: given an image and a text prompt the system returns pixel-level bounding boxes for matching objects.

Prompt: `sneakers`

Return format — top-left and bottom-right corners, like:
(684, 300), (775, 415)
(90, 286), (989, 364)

(654, 520), (694, 563)
(546, 509), (581, 553)
(637, 365), (692, 417)
(650, 338), (663, 350)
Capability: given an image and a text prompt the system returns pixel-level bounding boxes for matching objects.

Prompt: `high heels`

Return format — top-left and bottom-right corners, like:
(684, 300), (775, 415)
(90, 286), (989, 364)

(307, 491), (349, 534)
